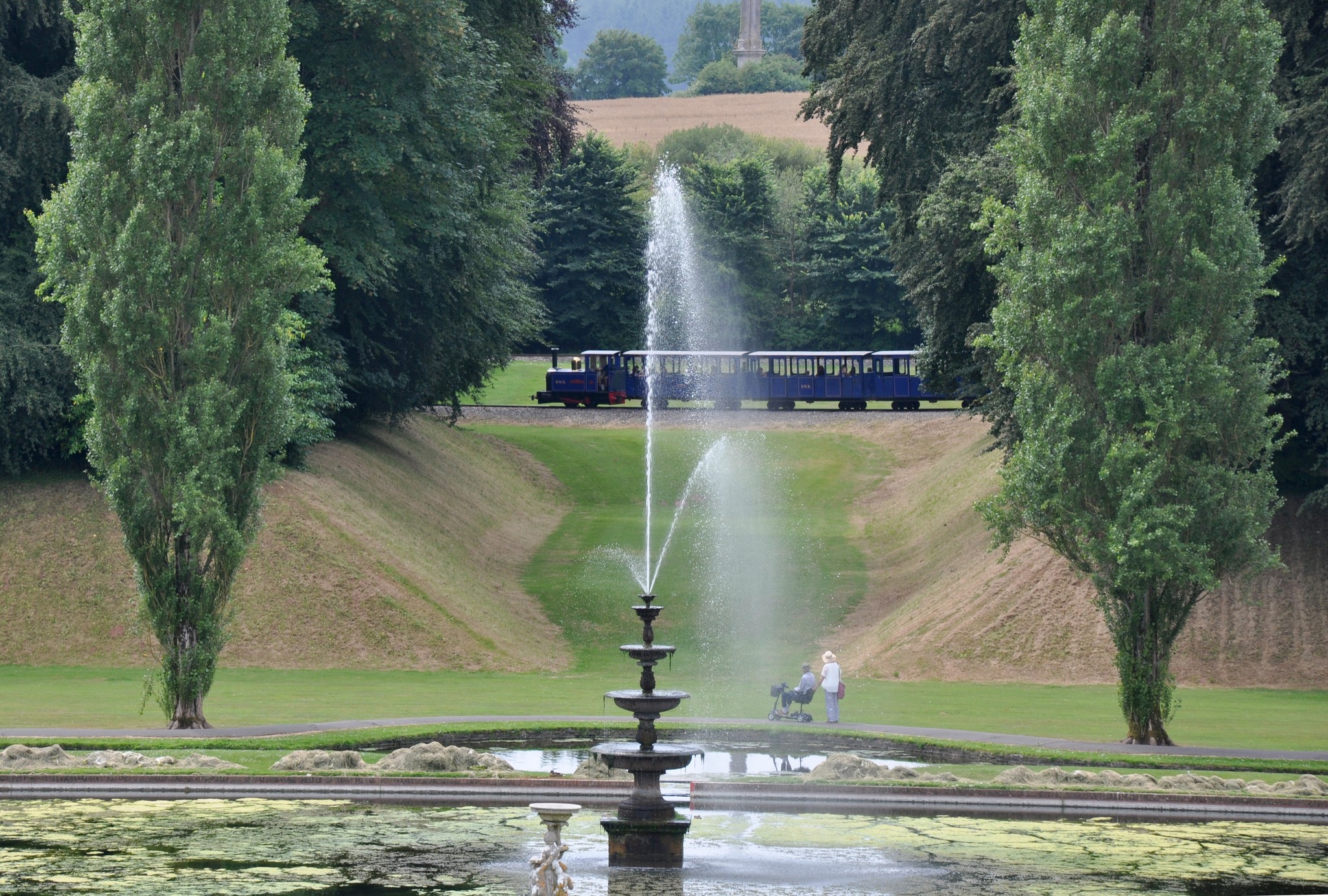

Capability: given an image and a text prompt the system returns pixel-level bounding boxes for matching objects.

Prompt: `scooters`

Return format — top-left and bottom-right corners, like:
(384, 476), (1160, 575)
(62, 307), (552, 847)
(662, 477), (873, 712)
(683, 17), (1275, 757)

(768, 683), (814, 723)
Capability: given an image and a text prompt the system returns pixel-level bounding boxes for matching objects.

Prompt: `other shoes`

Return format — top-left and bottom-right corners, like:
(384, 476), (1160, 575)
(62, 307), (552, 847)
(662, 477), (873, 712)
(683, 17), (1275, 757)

(775, 709), (789, 716)
(825, 720), (838, 723)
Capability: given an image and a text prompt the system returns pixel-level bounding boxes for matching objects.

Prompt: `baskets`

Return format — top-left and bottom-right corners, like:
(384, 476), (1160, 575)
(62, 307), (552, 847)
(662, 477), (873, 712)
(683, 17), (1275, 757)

(770, 685), (781, 697)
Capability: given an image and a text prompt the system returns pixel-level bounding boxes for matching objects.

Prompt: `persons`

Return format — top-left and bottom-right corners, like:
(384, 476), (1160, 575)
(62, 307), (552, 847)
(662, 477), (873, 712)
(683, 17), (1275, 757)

(741, 362), (750, 372)
(815, 365), (825, 376)
(812, 650), (842, 723)
(755, 367), (767, 377)
(775, 662), (816, 717)
(632, 365), (646, 376)
(839, 364), (855, 376)
(596, 358), (615, 389)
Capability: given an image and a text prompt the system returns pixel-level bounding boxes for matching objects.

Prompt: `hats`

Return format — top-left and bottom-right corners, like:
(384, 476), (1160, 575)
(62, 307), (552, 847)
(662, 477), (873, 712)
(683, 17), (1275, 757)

(802, 663), (811, 670)
(822, 650), (836, 663)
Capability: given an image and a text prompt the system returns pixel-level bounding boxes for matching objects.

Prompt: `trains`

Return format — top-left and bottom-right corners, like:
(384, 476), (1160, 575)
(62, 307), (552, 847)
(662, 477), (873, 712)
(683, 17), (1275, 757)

(530, 348), (983, 410)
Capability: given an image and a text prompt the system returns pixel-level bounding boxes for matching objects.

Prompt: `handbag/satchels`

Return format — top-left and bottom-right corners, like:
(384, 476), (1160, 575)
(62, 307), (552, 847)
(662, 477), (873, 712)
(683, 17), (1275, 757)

(837, 681), (845, 699)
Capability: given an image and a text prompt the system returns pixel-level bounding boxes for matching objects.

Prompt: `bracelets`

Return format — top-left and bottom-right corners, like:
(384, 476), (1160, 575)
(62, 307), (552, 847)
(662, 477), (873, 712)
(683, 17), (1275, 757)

(816, 685), (819, 687)
